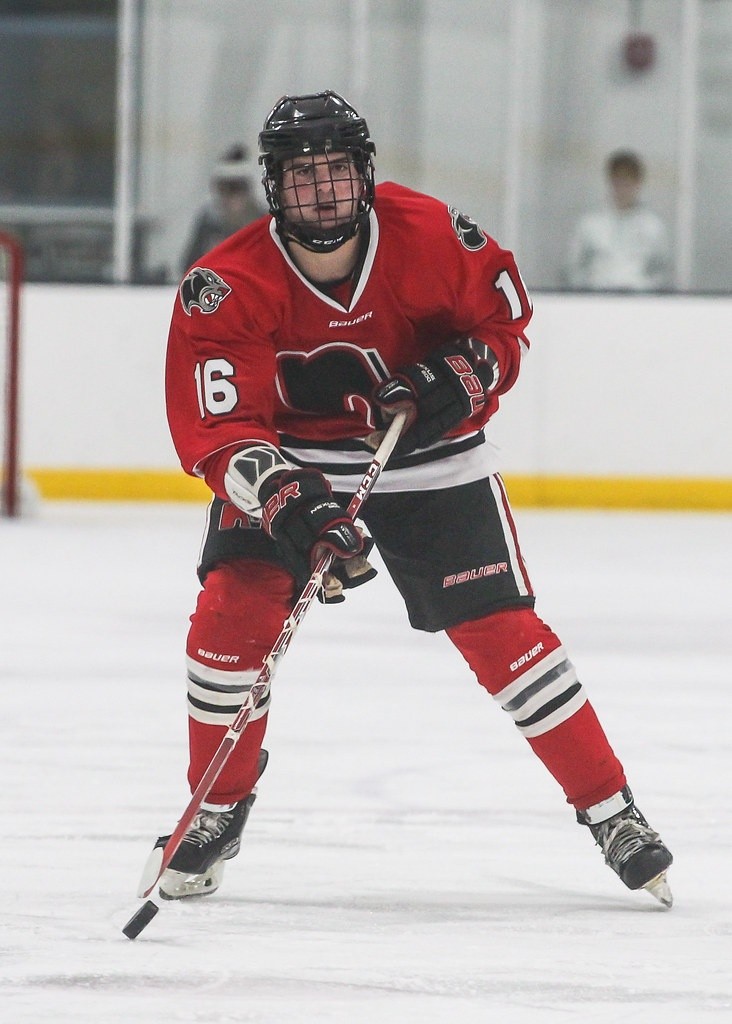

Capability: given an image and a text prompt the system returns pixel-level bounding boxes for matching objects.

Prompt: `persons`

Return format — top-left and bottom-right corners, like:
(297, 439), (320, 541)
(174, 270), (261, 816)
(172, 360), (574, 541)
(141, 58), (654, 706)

(178, 141), (267, 277)
(562, 150), (672, 289)
(154, 87), (673, 902)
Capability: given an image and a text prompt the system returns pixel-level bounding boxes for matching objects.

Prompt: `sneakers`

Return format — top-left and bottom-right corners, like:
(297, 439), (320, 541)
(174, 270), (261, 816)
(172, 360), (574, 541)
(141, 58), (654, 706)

(576, 782), (675, 909)
(160, 749), (271, 899)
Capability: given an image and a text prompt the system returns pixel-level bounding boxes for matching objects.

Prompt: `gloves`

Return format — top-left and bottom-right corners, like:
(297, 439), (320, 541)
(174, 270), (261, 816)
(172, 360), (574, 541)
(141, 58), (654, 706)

(364, 341), (494, 456)
(260, 469), (379, 603)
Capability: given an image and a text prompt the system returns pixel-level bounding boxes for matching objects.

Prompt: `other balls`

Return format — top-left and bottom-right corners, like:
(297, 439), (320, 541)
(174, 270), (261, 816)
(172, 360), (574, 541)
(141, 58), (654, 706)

(122, 899), (160, 940)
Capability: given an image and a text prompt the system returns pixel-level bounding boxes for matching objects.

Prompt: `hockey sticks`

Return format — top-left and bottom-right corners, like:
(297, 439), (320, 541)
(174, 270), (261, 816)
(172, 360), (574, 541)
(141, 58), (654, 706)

(134, 411), (407, 902)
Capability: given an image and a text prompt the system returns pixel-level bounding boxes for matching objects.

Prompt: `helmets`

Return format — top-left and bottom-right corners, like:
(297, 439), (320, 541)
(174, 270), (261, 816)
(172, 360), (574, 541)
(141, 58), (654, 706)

(258, 91), (379, 253)
